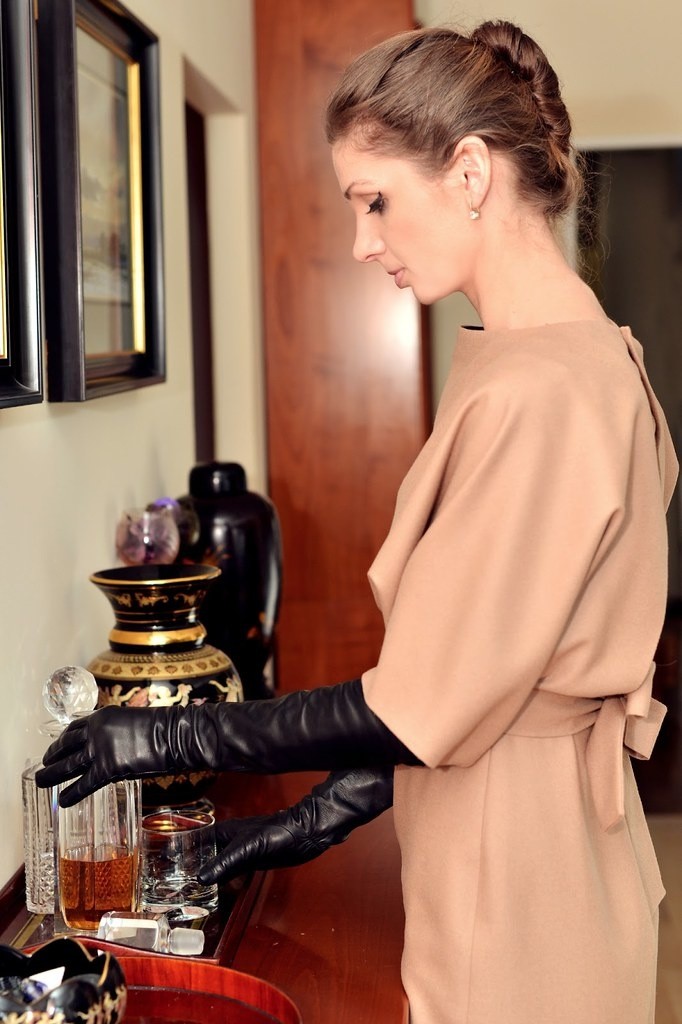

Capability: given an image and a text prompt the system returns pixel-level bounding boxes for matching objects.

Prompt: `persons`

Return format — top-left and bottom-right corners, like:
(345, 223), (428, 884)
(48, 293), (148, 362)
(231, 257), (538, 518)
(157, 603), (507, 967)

(31, 19), (682, 1024)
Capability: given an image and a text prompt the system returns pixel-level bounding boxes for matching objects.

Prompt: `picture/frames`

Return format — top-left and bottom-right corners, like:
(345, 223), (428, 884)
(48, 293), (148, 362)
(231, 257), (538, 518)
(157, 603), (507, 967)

(0, 0), (45, 410)
(35, 0), (168, 403)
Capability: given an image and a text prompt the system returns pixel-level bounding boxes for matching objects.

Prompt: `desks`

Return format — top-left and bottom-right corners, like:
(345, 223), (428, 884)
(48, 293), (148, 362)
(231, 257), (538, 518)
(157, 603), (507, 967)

(0, 598), (413, 1024)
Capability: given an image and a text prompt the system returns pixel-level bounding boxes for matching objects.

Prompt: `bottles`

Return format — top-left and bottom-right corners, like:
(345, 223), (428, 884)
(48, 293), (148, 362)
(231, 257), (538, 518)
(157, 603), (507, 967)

(16, 668), (120, 914)
(94, 909), (205, 955)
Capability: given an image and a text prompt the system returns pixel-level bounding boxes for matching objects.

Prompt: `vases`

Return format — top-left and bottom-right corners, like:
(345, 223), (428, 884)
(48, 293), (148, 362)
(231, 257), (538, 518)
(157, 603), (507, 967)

(85, 561), (246, 849)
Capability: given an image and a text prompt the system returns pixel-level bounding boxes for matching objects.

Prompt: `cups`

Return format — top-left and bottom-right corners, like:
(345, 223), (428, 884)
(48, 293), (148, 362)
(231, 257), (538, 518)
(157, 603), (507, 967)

(52, 768), (142, 932)
(140, 814), (218, 915)
